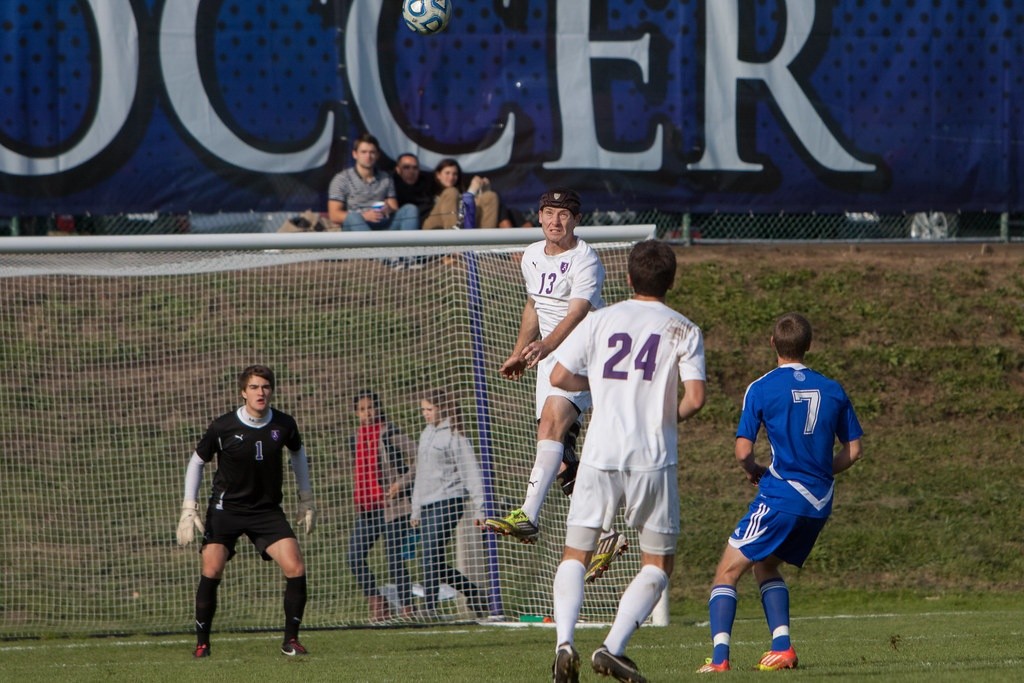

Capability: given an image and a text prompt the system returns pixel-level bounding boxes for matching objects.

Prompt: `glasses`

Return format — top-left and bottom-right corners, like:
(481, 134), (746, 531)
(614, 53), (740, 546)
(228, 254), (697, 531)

(399, 164), (417, 169)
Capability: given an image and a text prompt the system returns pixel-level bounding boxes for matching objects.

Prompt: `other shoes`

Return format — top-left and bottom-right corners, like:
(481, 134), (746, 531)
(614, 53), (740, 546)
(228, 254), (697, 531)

(400, 605), (418, 619)
(368, 597), (392, 621)
(416, 609), (445, 623)
(475, 596), (495, 620)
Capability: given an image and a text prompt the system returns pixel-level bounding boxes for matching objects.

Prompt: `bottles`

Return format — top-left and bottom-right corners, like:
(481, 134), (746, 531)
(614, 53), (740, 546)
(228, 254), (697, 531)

(520, 615), (551, 623)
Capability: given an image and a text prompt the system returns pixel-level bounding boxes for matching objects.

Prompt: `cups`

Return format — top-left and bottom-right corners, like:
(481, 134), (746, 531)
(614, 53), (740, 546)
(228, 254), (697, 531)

(372, 202), (385, 220)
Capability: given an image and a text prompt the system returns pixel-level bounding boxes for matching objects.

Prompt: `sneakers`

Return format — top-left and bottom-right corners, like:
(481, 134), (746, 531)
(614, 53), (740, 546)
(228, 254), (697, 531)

(279, 640), (306, 656)
(751, 647), (798, 671)
(194, 645), (209, 656)
(486, 507), (539, 546)
(696, 658), (730, 674)
(584, 532), (630, 581)
(552, 642), (580, 683)
(591, 645), (647, 683)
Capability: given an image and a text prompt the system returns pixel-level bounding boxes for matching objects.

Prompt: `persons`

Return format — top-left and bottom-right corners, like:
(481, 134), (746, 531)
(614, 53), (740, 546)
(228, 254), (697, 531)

(176, 365), (316, 657)
(549, 238), (707, 683)
(408, 389), (490, 619)
(483, 185), (628, 583)
(328, 133), (419, 267)
(394, 153), (533, 229)
(697, 312), (864, 672)
(346, 388), (417, 620)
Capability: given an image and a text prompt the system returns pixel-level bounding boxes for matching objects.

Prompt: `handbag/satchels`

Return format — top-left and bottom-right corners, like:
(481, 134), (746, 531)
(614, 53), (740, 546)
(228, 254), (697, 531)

(279, 211), (329, 232)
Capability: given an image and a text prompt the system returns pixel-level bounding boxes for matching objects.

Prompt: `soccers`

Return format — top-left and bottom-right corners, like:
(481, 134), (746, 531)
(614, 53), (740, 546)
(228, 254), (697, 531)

(400, 0), (454, 35)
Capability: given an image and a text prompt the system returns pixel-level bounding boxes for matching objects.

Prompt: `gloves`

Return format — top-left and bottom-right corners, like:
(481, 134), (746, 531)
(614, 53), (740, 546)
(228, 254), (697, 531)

(296, 490), (318, 535)
(177, 502), (204, 546)
